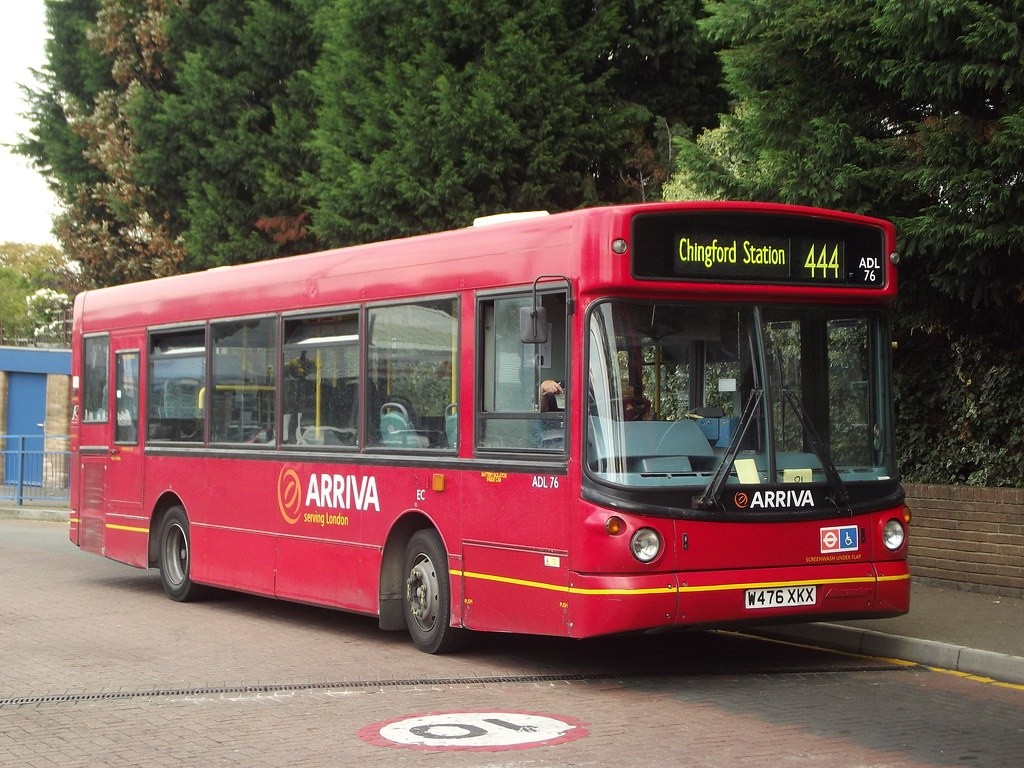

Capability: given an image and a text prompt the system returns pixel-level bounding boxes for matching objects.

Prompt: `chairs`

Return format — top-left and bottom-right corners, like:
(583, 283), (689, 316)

(101, 384), (175, 441)
(379, 404), (430, 449)
(385, 395), (421, 432)
(163, 376), (205, 441)
(445, 403), (459, 449)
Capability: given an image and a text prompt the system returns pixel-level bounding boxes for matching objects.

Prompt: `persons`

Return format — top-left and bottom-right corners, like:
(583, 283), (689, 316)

(537, 379), (564, 415)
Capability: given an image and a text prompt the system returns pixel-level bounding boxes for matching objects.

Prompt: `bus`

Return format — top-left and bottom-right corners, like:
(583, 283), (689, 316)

(69, 200), (912, 656)
